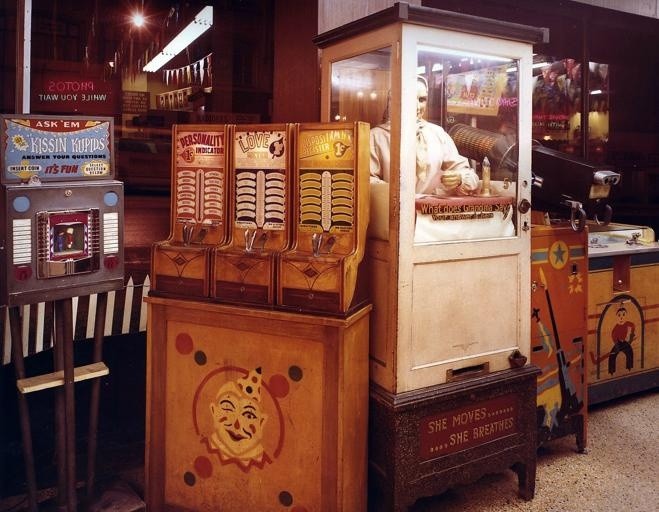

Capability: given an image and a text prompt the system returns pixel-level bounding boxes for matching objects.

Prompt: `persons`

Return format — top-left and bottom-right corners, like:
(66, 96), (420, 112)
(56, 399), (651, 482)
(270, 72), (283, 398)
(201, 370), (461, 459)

(607, 306), (635, 378)
(368, 74), (459, 241)
(200, 367), (272, 471)
